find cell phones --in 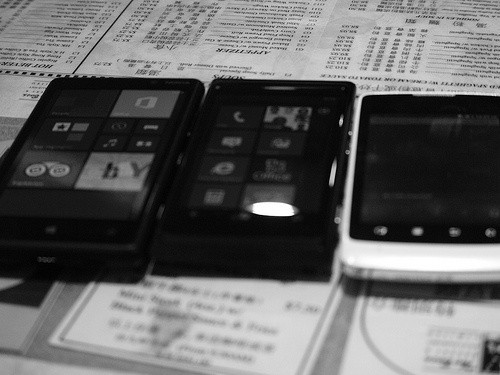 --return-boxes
[0,75,204,282]
[338,90,500,284]
[151,78,356,282]
